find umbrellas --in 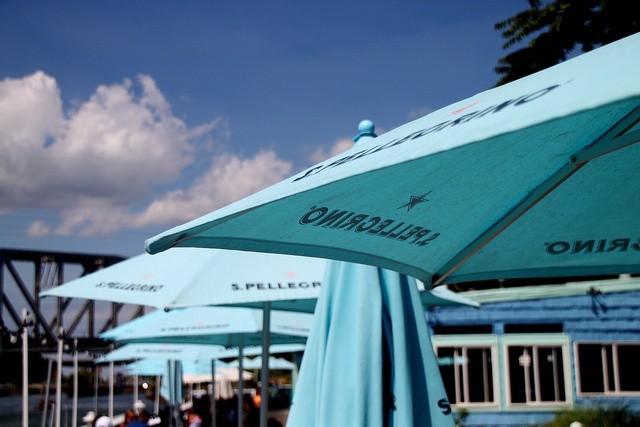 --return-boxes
[94,343,306,426]
[159,360,184,426]
[284,118,456,425]
[122,357,255,427]
[97,306,315,427]
[41,248,481,427]
[145,27,640,292]
